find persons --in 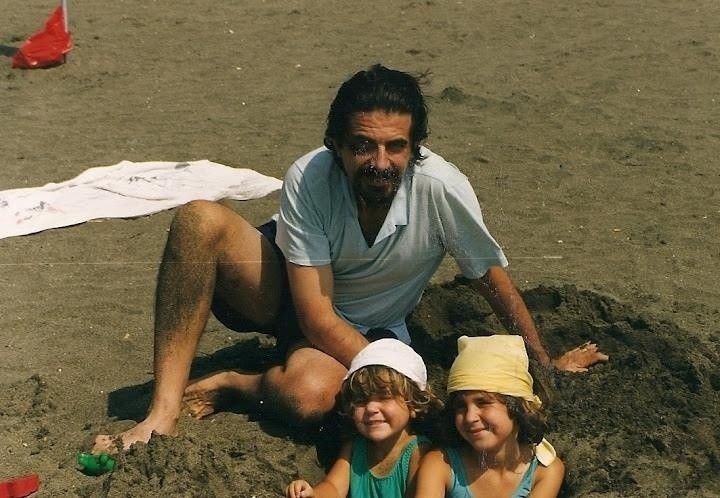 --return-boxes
[285,339,435,498]
[414,335,565,498]
[91,63,609,458]
